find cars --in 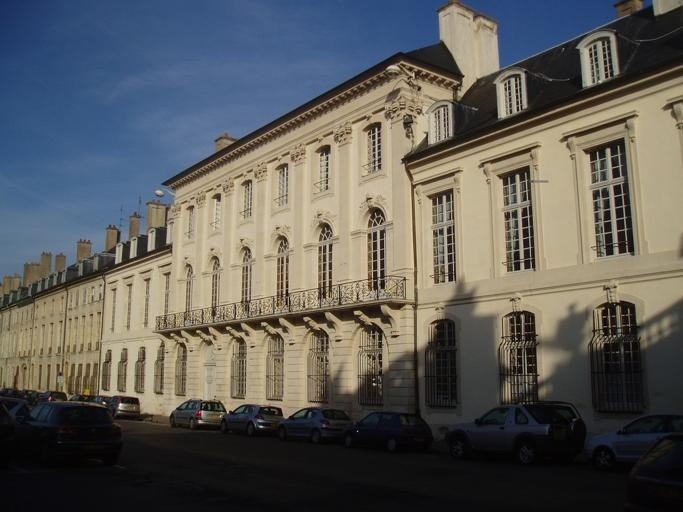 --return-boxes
[585,412,683,511]
[344,411,433,452]
[0,387,141,466]
[220,404,285,436]
[169,400,227,430]
[444,400,587,464]
[276,407,354,444]
